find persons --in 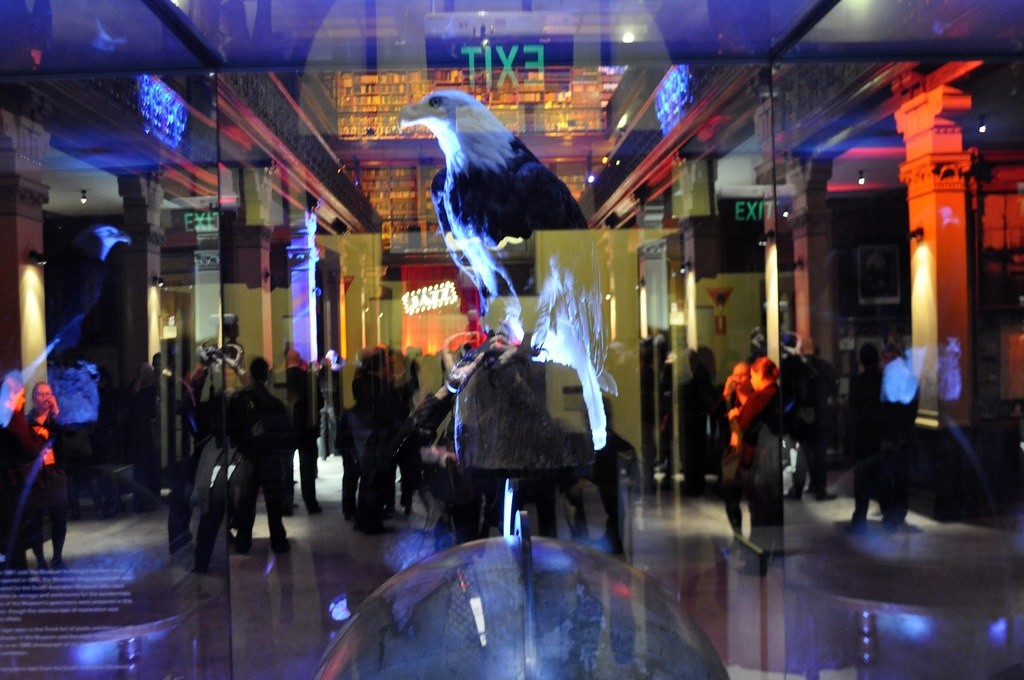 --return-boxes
[89,343,639,573]
[0,378,50,570]
[270,347,324,514]
[25,382,69,570]
[225,356,294,552]
[842,343,904,537]
[637,324,921,576]
